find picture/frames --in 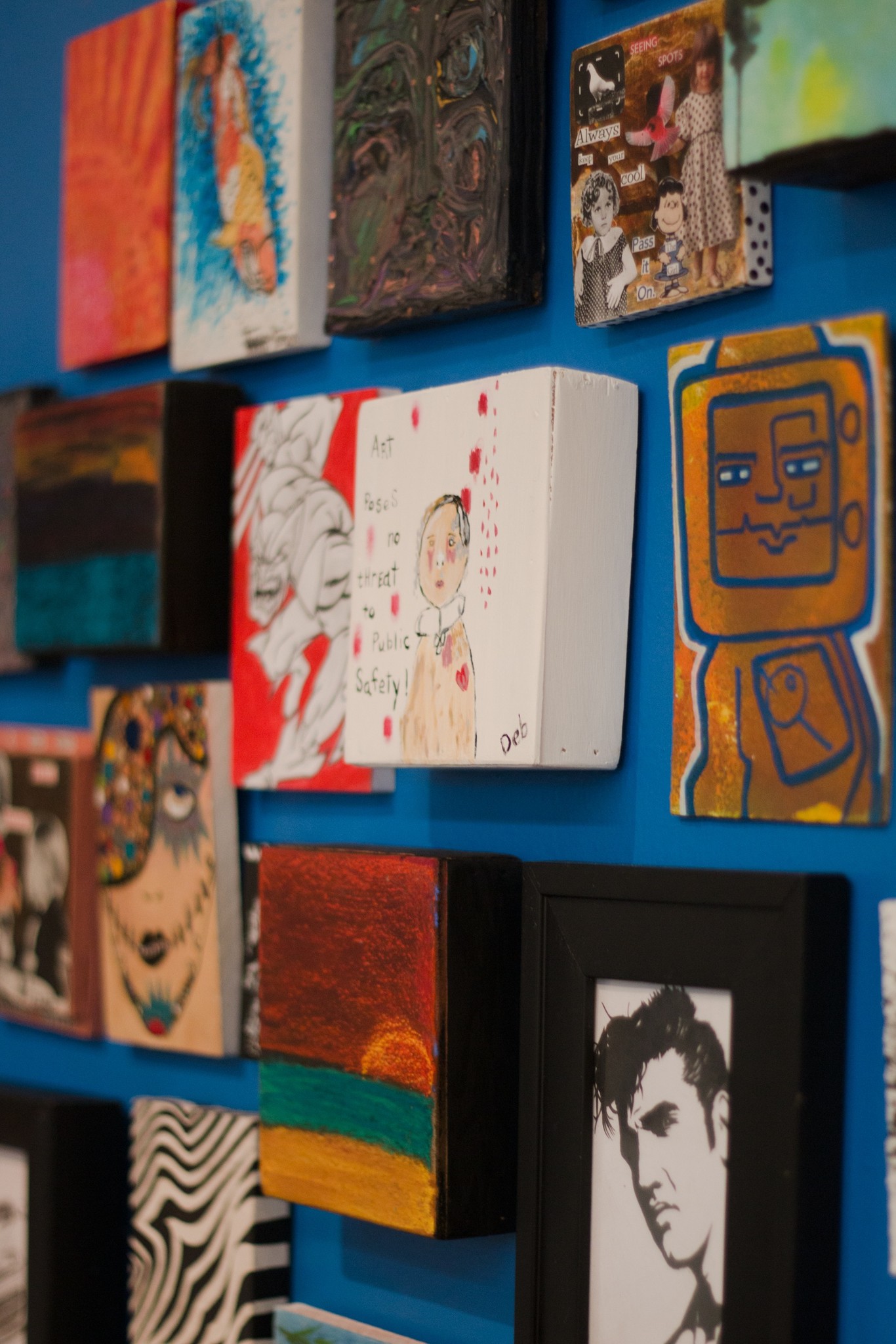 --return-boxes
[0,726,100,1039]
[523,859,855,1342]
